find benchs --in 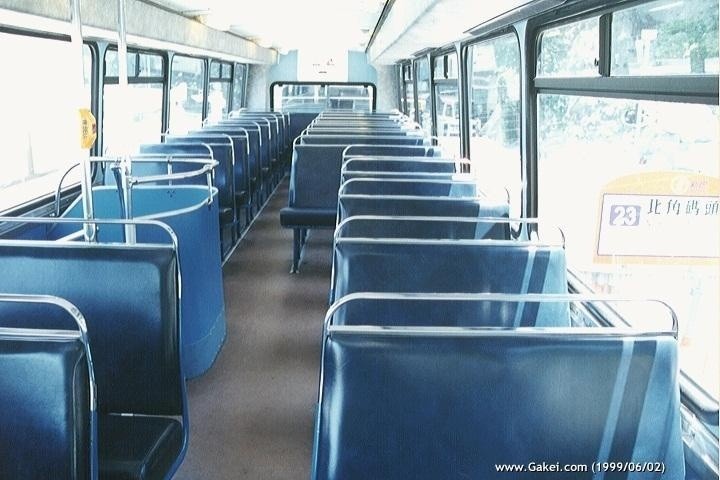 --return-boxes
[337,178,512,241]
[101,109,290,255]
[340,156,474,181]
[0,216,189,478]
[0,289,101,479]
[334,108,440,146]
[327,214,576,333]
[310,291,690,480]
[342,145,446,156]
[280,108,334,275]
